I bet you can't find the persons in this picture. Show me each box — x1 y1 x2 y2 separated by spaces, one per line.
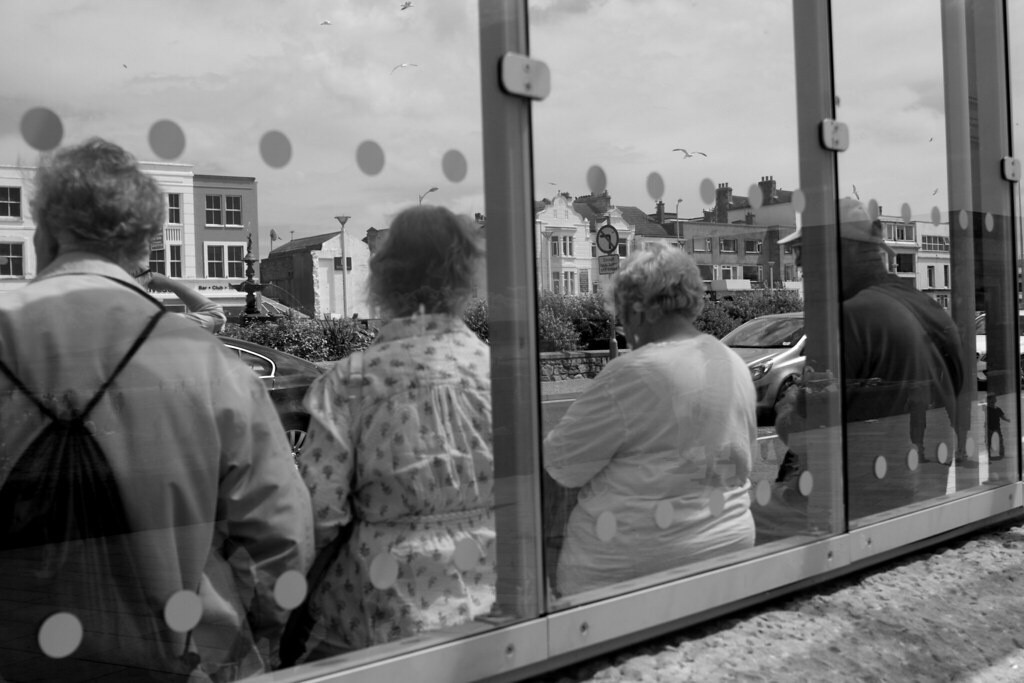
894 332 1011 462
747 200 965 545
542 240 761 597
272 203 496 674
0 135 315 683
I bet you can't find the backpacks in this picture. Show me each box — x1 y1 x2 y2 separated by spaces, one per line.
0 308 183 683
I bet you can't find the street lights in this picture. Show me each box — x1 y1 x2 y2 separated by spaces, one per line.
678 240 686 249
675 199 682 248
766 262 775 299
334 216 352 318
418 186 438 205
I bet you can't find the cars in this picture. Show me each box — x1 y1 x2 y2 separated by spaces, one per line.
217 334 328 471
976 311 1024 390
719 311 807 426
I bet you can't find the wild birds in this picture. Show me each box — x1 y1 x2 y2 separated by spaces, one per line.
123 1 708 185
852 137 939 201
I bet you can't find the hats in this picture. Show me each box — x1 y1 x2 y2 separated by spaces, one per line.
777 197 885 247
